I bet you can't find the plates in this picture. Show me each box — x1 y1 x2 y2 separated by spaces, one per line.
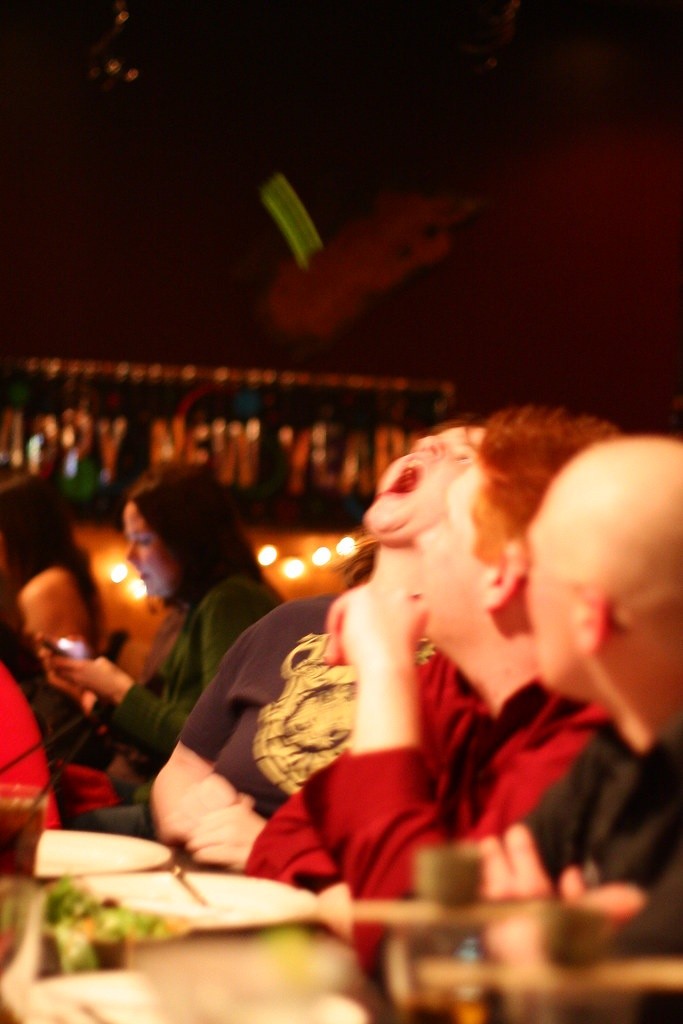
82 871 317 933
32 829 172 881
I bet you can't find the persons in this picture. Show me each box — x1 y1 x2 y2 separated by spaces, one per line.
1 467 280 864
472 433 682 1024
149 409 494 869
244 412 622 971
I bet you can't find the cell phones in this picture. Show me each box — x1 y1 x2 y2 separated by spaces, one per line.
42 638 65 658
